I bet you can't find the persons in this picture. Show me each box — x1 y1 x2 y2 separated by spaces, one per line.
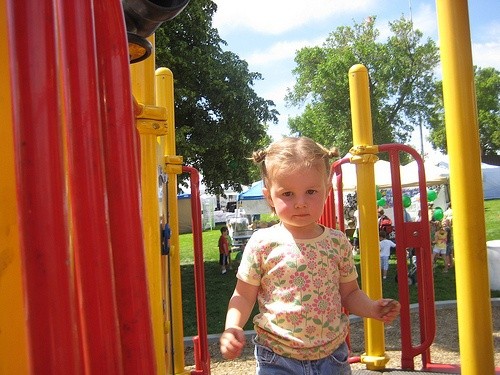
431 202 454 273
218 227 233 275
219 137 400 375
378 229 397 280
377 207 393 239
351 204 360 256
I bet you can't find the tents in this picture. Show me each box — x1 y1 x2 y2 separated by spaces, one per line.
374 152 500 211
328 153 402 221
237 180 269 213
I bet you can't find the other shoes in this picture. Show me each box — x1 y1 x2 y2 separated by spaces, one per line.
222 269 227 274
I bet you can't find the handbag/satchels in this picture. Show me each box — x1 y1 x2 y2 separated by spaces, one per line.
380 217 393 233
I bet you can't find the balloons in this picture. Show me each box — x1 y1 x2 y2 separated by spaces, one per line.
375 185 387 207
433 208 443 220
402 195 411 208
427 189 437 201
